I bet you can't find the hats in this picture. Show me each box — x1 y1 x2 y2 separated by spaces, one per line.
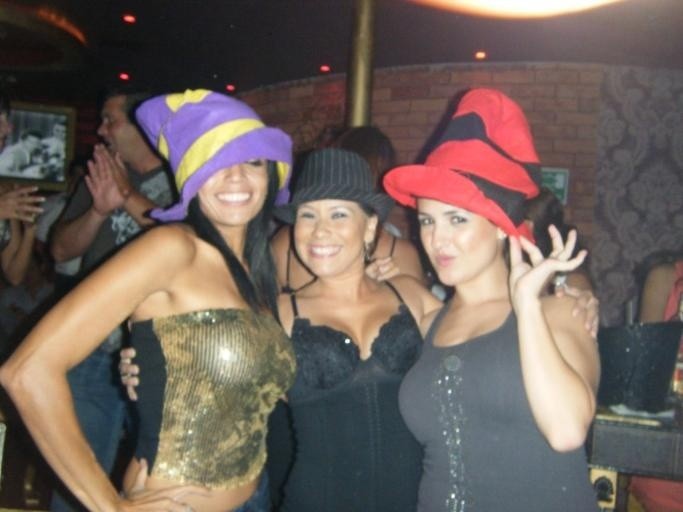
129 87 291 226
267 146 395 224
379 83 543 253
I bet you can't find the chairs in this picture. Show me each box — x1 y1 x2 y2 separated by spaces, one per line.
587 251 683 512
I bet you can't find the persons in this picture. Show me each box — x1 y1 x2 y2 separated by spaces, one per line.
628 257 683 512
0 87 601 510
